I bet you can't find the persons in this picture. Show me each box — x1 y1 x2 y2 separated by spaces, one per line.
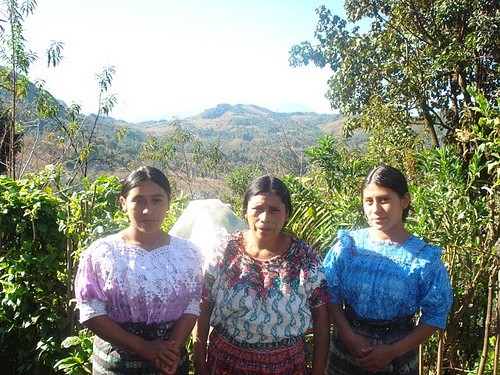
322 165 454 375
194 175 331 375
74 166 204 375
